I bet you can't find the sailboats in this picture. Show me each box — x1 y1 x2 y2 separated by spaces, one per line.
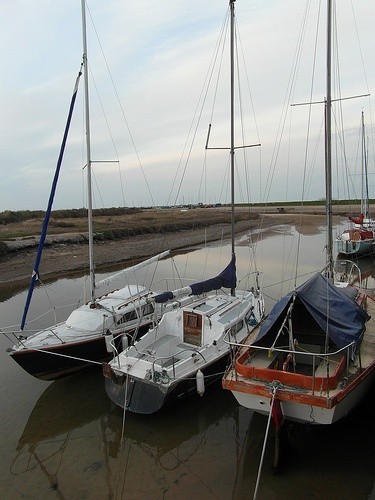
0 0 189 380
217 0 375 424
86 0 265 414
334 110 374 256
